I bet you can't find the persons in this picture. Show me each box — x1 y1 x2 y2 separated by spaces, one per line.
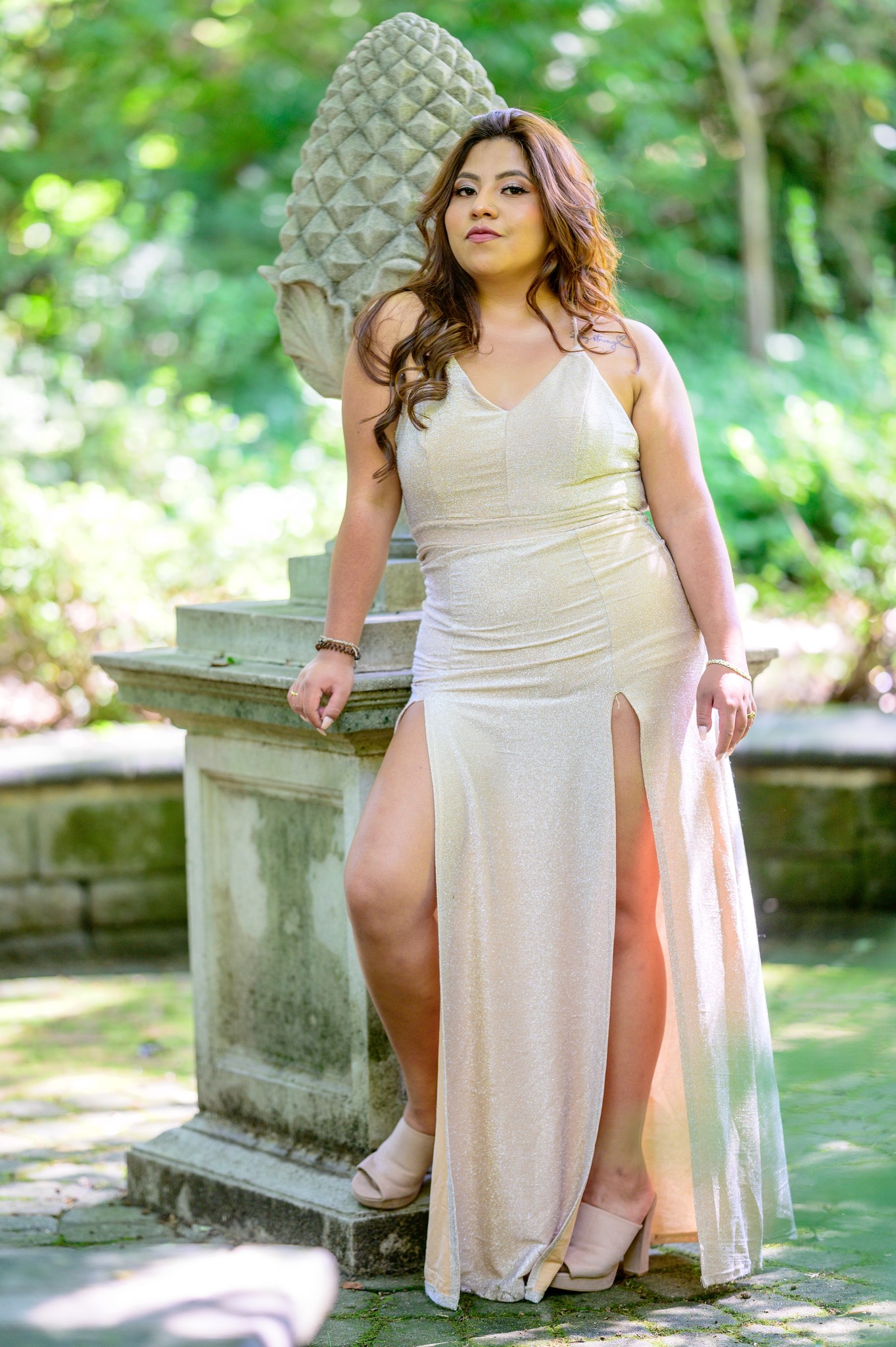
289 103 794 1306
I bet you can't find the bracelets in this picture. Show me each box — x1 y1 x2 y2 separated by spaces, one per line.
705 657 755 682
313 636 364 665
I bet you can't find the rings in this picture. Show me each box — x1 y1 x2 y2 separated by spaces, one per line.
744 710 759 721
292 691 301 698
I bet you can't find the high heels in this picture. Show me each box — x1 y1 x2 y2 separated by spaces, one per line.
351 1115 436 1209
552 1192 657 1292
